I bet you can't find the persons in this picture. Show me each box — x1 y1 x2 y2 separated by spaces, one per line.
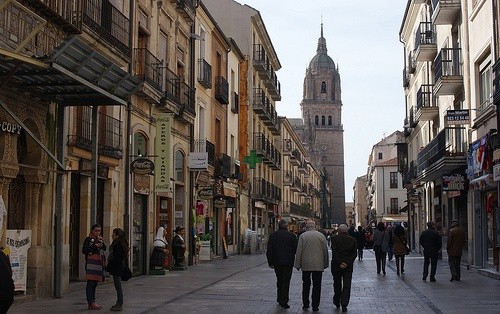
297 223 365 262
403 222 410 247
0 194 15 314
386 222 393 261
331 224 358 312
266 220 298 308
419 221 442 282
294 221 329 312
172 225 186 267
105 228 130 311
153 226 169 270
445 218 465 282
391 225 407 275
82 224 107 310
373 221 390 275
225 212 233 245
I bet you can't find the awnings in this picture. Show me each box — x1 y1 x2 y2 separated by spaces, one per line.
399 204 414 212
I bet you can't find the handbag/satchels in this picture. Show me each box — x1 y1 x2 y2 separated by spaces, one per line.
374 244 382 252
121 268 132 281
405 247 409 255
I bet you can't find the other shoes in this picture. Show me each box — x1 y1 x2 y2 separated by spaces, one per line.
422 277 426 280
430 278 437 282
110 305 123 311
456 278 460 281
342 307 347 312
333 300 340 308
397 272 399 275
278 301 290 308
377 272 380 274
383 271 386 274
88 303 102 310
313 307 320 311
450 274 457 282
401 270 404 273
303 305 309 309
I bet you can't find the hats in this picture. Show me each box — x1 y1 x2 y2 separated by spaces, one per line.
451 219 458 222
